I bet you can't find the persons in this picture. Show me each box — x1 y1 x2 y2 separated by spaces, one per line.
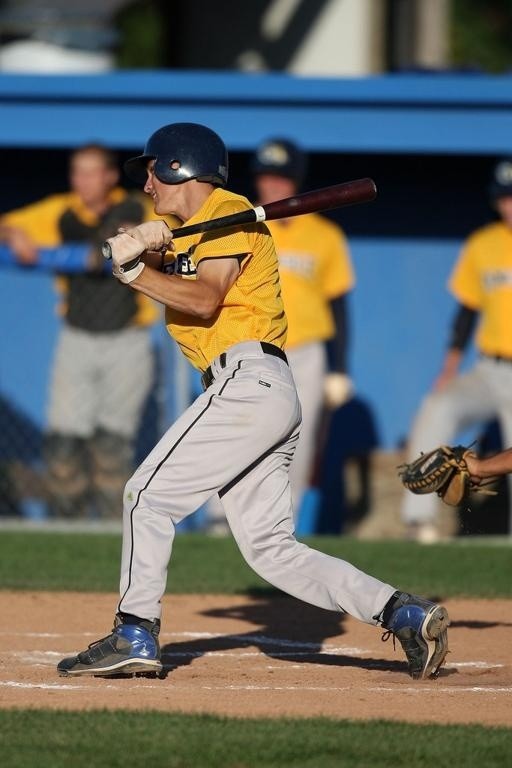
253 137 356 528
0 143 181 523
56 123 451 681
462 446 512 485
403 163 512 545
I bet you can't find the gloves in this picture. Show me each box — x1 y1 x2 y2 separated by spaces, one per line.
106 220 176 285
325 373 354 412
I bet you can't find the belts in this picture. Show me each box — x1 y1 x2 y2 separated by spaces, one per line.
200 341 288 391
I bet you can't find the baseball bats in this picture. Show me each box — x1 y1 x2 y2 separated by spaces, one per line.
101 176 377 259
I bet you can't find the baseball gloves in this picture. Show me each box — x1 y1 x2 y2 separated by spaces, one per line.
402 445 482 506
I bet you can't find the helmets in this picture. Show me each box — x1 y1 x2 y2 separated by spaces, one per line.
489 159 512 195
127 122 231 185
250 137 307 182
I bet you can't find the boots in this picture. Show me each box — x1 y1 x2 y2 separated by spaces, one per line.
57 614 165 678
376 590 449 681
